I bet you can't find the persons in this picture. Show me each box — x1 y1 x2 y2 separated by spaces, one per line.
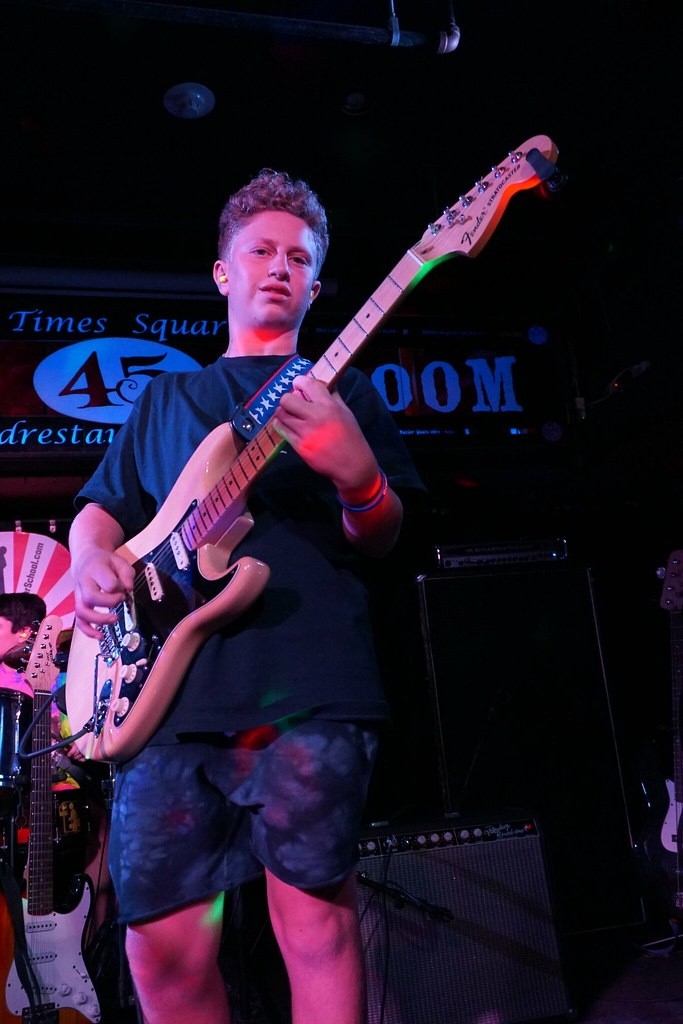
0 593 109 898
69 168 403 1024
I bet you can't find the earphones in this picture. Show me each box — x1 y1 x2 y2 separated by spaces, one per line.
218 276 227 283
310 291 314 297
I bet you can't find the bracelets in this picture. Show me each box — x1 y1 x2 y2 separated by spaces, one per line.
337 469 387 512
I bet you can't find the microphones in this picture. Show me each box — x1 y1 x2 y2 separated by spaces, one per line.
427 905 455 923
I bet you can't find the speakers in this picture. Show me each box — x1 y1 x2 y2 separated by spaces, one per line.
420 563 646 941
356 816 571 1024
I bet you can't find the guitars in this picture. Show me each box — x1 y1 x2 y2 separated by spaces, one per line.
4 609 117 1022
62 126 571 767
